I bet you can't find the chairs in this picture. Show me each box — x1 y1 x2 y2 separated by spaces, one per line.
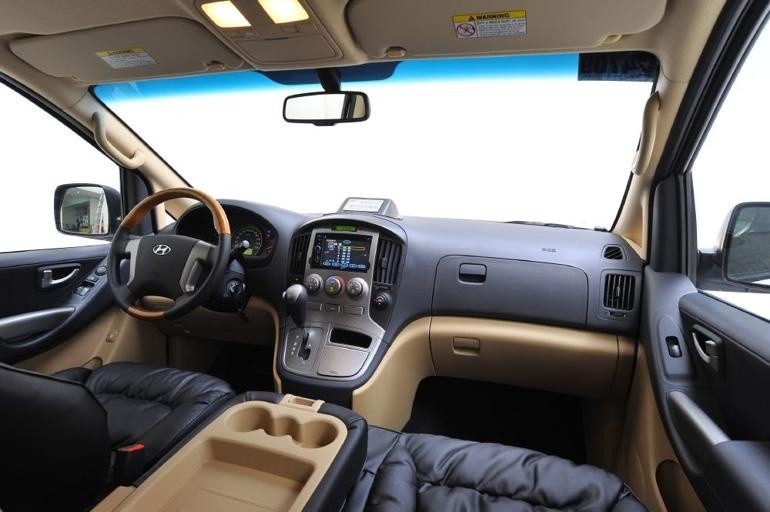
345 427 653 511
0 354 234 511
